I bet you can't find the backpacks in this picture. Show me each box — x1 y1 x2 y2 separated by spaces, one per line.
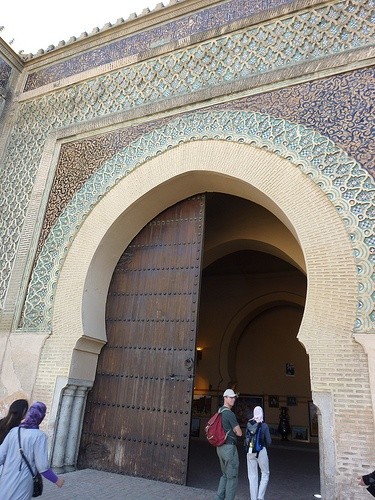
205 408 232 446
244 422 265 452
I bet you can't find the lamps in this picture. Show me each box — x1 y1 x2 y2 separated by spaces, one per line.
197 351 202 365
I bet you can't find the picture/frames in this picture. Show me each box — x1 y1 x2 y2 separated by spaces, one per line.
234 395 265 428
268 423 282 440
190 418 200 437
308 400 318 437
268 395 279 408
290 425 308 440
287 396 298 406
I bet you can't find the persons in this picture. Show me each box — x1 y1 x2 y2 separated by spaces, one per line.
246 406 272 500
0 399 65 500
357 470 375 498
216 389 242 500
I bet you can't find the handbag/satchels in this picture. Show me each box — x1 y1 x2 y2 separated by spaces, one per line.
33 473 43 497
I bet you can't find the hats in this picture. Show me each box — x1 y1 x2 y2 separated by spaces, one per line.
224 389 238 398
